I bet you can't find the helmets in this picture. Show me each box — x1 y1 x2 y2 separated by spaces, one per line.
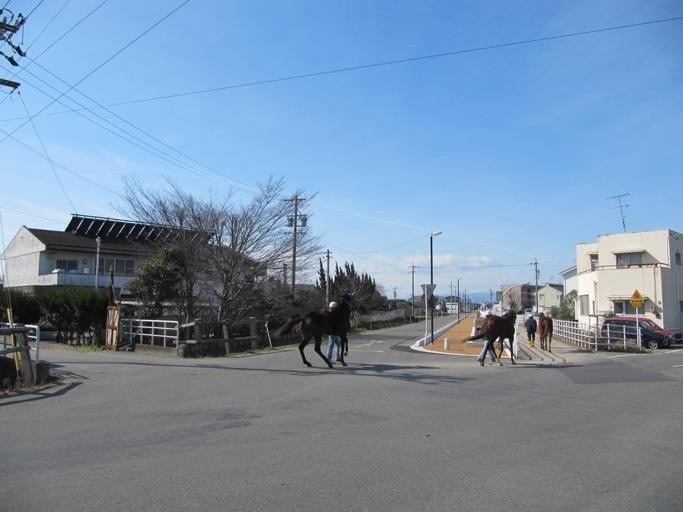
486 311 492 315
528 314 533 317
329 302 338 308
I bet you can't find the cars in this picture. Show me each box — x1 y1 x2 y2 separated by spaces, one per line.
601 316 676 350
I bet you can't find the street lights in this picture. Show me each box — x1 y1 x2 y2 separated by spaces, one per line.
429 230 442 344
456 276 464 324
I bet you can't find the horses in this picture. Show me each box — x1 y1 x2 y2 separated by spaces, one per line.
274 291 355 368
460 309 517 366
537 312 554 352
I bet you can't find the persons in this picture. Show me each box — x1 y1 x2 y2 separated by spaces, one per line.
476 310 501 367
523 315 537 348
325 301 344 365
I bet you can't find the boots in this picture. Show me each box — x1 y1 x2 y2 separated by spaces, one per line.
528 340 534 348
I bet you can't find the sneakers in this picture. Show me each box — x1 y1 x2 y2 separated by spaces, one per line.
329 360 335 364
335 357 341 361
